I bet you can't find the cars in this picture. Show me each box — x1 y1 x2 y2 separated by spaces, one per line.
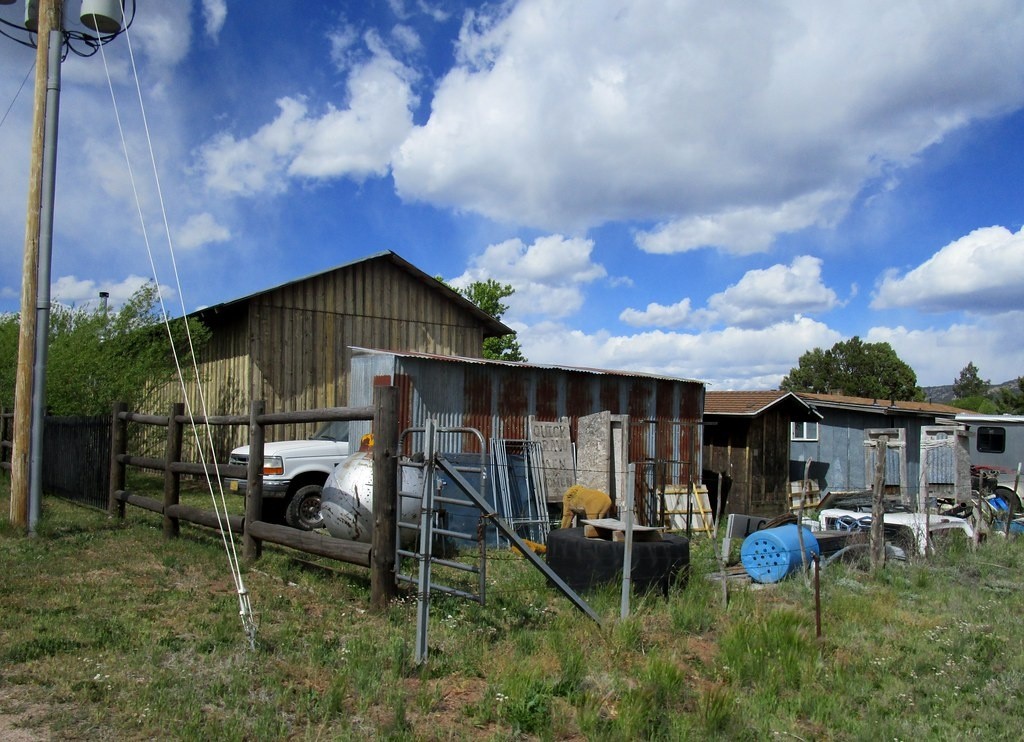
224 421 351 531
